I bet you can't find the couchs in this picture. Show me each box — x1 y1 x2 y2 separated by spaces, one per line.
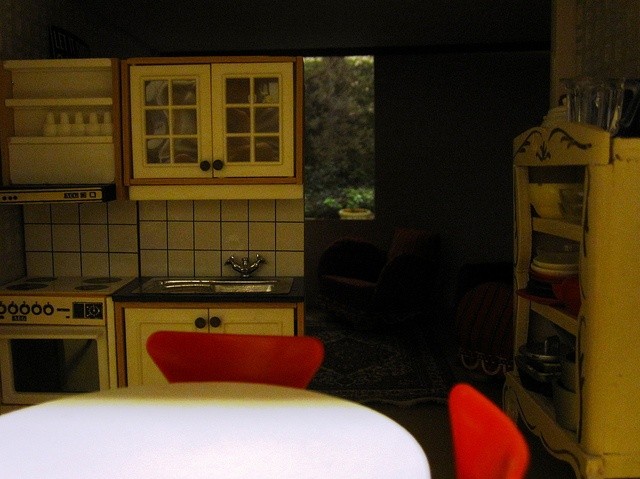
317 226 441 329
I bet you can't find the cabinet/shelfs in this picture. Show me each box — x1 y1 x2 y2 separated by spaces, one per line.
502 122 640 479
114 302 305 389
3 58 116 184
120 55 304 187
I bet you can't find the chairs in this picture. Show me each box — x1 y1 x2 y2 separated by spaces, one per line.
447 383 531 479
146 331 325 389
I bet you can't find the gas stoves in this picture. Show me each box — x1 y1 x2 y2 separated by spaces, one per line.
0 274 130 327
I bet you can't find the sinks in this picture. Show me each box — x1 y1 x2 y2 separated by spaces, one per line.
129 278 212 294
214 277 294 294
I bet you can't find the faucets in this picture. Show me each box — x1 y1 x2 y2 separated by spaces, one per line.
240 258 252 276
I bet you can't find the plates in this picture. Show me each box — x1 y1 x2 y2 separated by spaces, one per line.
530 253 583 280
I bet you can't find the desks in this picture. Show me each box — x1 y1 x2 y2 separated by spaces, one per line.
0 382 432 479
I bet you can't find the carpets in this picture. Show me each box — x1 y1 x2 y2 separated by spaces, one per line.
304 319 493 407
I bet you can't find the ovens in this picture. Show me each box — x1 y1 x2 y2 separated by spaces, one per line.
1 324 110 407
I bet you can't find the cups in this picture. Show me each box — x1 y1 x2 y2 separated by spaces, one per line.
559 75 639 137
